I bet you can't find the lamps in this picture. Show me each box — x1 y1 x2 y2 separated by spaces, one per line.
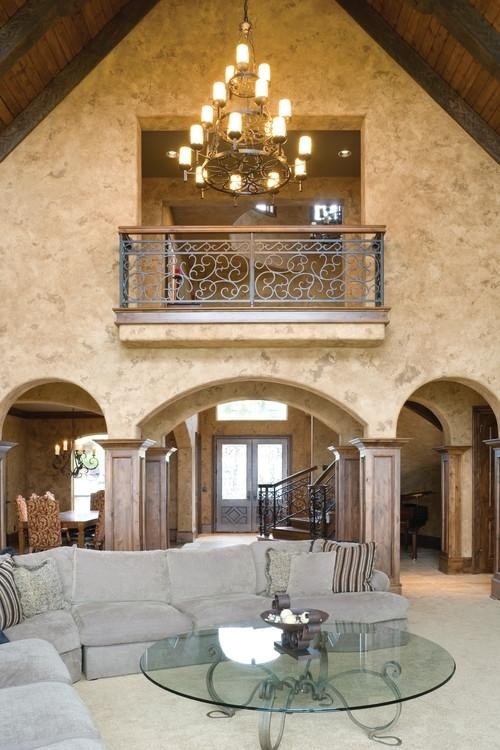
177 0 319 205
51 408 98 480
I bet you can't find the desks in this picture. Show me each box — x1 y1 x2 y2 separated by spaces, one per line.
139 624 456 747
14 484 116 554
